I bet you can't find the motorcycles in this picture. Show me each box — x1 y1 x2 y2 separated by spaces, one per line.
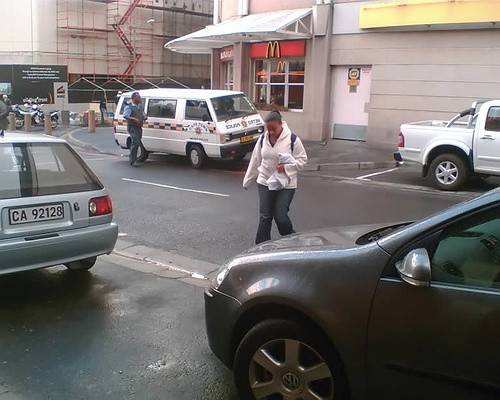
7 104 43 129
28 106 59 129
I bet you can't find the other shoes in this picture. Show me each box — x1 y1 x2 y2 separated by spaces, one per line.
131 162 140 167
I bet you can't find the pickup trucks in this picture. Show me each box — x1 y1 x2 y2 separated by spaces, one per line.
394 99 500 191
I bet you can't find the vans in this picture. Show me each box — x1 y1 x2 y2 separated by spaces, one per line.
112 88 267 169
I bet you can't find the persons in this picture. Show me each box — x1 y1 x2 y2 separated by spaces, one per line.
123 92 147 167
0 94 11 135
100 91 122 126
160 103 175 118
242 111 308 245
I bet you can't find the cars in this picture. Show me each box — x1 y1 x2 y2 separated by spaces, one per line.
0 130 119 277
203 186 500 399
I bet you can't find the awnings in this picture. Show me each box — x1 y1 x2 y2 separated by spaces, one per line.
163 9 313 54
